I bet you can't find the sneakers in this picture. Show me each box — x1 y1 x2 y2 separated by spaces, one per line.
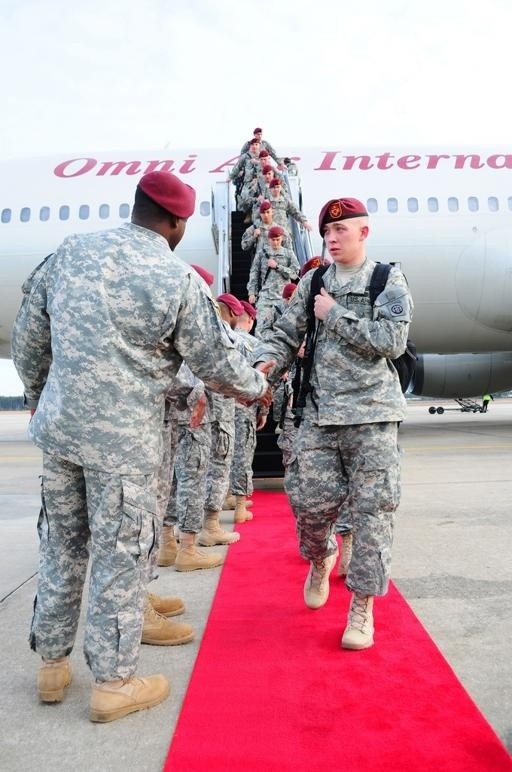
36 655 73 705
147 589 187 618
88 675 169 724
139 601 196 646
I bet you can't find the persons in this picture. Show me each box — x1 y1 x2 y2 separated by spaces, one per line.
11 169 272 721
139 264 268 646
229 128 313 344
482 394 493 411
253 197 414 649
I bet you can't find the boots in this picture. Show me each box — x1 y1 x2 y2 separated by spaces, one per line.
302 552 337 610
198 508 240 546
174 530 226 571
234 494 253 524
340 590 375 649
275 421 283 436
338 534 353 576
157 525 179 567
221 490 253 511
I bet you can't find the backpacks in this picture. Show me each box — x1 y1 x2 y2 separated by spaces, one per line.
309 262 417 394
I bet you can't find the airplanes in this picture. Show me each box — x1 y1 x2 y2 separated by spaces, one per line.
0 140 512 482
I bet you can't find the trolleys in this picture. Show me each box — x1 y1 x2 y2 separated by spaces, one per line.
427 397 488 415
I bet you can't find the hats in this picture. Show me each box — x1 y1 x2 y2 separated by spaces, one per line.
262 165 274 176
318 197 368 238
190 264 214 286
139 170 195 219
259 202 271 214
250 138 260 145
282 283 296 299
216 294 243 316
258 150 269 158
268 226 284 239
269 178 281 189
239 300 256 320
253 128 263 134
300 256 331 279
